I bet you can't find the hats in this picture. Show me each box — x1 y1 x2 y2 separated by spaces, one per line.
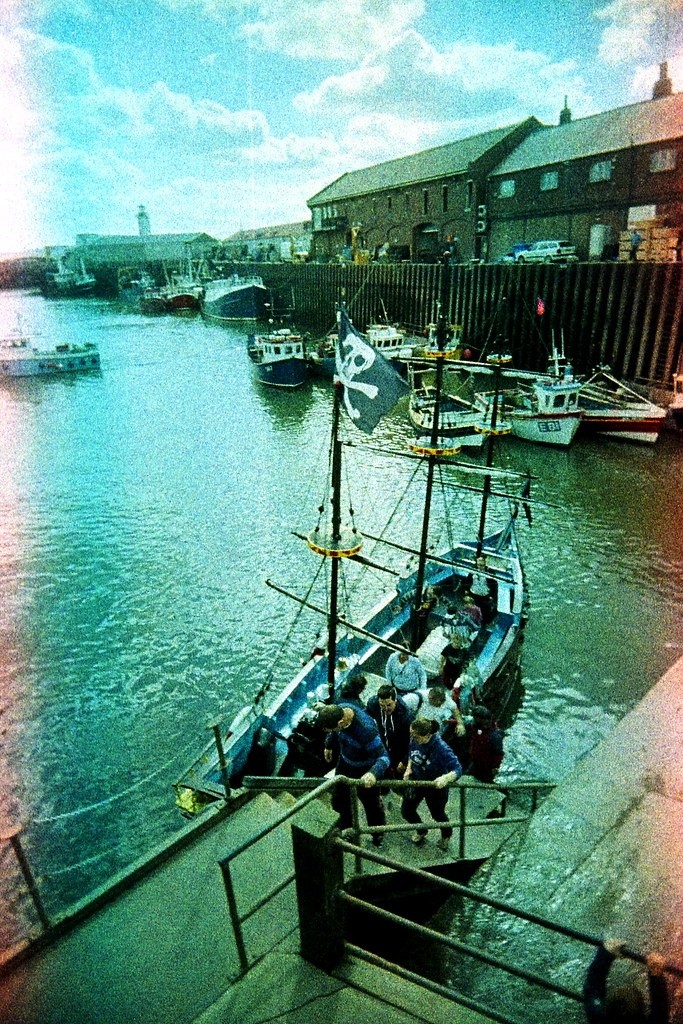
460 674 475 689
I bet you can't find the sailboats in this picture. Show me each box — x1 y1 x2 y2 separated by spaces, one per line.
170 251 531 802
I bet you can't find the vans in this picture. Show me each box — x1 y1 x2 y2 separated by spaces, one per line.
280 242 309 263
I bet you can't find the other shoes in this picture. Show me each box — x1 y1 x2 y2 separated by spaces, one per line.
411 831 425 843
333 819 351 831
372 834 383 847
439 836 450 849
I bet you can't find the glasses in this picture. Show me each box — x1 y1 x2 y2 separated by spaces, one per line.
463 600 470 604
477 564 485 566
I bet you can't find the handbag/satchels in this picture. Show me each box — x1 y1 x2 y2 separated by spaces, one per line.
406 774 418 798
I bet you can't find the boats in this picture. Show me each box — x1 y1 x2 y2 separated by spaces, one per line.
576 366 665 447
0 334 102 379
363 323 462 362
115 257 268 321
39 253 97 297
473 347 585 449
658 374 683 432
408 366 514 449
244 300 339 390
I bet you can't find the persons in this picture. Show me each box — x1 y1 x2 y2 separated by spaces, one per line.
317 702 390 848
400 718 462 850
629 227 643 263
366 583 503 789
466 556 496 630
441 234 458 264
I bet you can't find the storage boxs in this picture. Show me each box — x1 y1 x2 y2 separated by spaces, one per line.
619 216 683 261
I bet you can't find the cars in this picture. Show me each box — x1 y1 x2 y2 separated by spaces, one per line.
514 239 578 264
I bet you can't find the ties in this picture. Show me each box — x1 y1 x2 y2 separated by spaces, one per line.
386 714 393 748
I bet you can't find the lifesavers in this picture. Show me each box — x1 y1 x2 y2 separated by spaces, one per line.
453 675 477 714
